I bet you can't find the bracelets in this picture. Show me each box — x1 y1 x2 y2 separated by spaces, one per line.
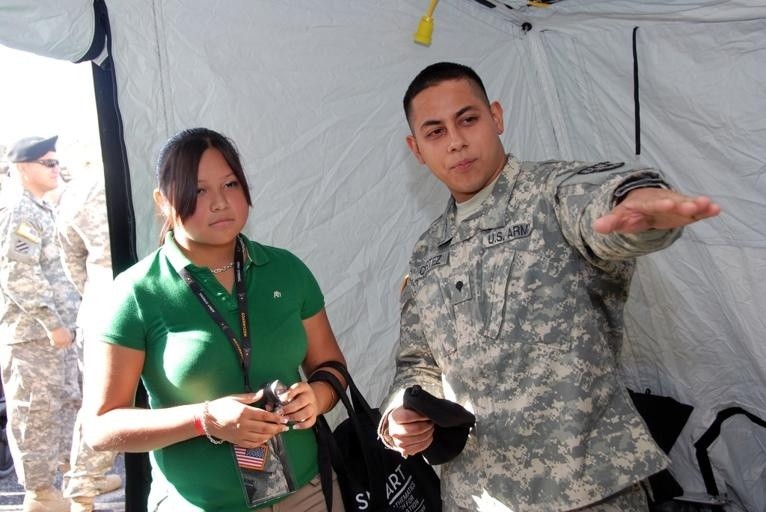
201 399 224 445
192 404 206 435
317 381 335 413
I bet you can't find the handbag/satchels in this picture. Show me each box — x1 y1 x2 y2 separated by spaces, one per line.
306 359 445 512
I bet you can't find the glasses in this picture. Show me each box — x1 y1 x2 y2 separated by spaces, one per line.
35 159 59 168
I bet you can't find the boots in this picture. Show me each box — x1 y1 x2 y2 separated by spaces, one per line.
22 484 94 512
61 472 122 499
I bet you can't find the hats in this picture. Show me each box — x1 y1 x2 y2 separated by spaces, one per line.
7 135 58 163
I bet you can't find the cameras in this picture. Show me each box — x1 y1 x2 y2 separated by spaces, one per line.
260 378 306 424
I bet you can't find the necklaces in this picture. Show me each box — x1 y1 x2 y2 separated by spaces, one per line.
209 235 246 275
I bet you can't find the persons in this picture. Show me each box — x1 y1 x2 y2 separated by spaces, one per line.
2 131 85 510
371 62 723 510
57 141 129 511
79 128 351 512
